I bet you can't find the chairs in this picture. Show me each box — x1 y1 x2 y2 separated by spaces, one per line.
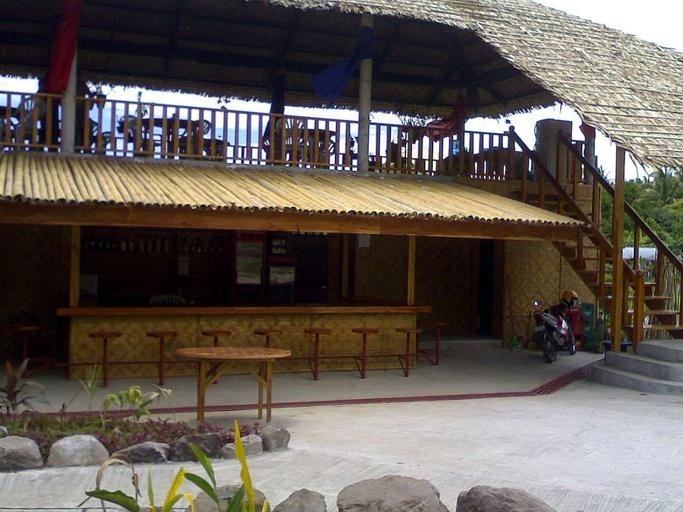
1 114 338 173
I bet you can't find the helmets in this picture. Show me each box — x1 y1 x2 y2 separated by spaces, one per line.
558 288 578 306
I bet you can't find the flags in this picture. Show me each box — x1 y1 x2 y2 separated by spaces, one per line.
39 1 80 109
311 25 374 103
421 94 466 141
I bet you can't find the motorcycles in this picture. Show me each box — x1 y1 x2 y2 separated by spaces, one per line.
531 300 576 364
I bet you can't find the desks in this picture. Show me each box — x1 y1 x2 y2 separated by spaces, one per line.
176 346 292 424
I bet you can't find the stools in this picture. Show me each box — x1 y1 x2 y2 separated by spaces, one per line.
253 329 282 382
146 330 178 386
352 328 379 379
421 318 447 365
202 329 231 384
19 325 42 376
89 331 122 387
304 328 332 380
397 327 423 377
